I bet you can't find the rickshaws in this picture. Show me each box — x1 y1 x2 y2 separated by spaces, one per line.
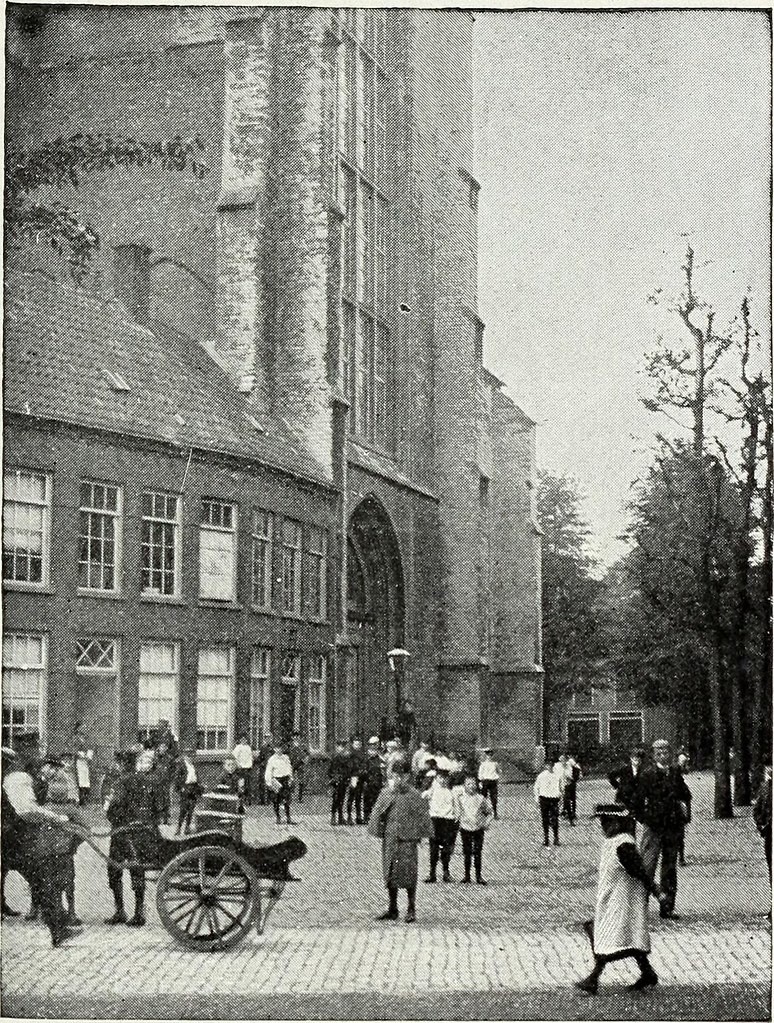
51 817 311 952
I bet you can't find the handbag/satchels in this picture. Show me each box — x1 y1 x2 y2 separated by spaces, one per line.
375 805 392 838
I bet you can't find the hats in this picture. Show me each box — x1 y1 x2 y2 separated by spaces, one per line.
391 759 412 774
651 738 669 749
544 755 553 763
11 730 40 749
367 735 380 744
589 802 630 818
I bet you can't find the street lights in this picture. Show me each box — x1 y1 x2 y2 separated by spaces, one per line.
385 643 412 741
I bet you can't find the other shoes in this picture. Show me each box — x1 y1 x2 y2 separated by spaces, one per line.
627 970 659 990
574 979 599 996
52 926 85 948
442 874 456 882
405 910 415 923
124 913 146 925
477 877 487 885
424 876 436 882
103 910 128 924
459 877 472 884
376 910 399 920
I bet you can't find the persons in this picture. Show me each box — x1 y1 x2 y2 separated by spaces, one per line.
532 752 584 845
330 737 500 922
103 752 154 924
752 769 773 921
121 720 309 837
1 720 93 946
575 737 692 994
